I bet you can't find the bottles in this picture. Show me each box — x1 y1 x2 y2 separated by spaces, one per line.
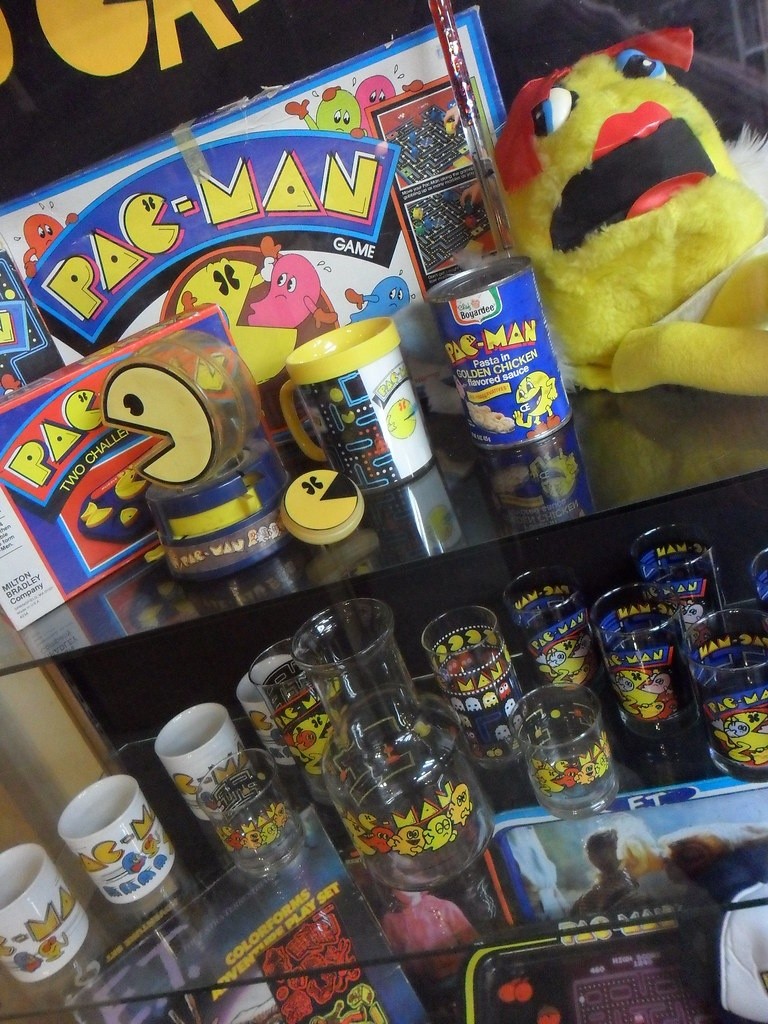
323 687 490 891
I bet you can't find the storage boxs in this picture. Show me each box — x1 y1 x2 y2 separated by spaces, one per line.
1 302 277 632
0 6 515 451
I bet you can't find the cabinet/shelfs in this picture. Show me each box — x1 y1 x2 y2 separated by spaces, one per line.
0 389 767 1023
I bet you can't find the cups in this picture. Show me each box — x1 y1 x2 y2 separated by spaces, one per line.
237 655 319 765
508 681 618 822
588 581 699 740
196 748 301 881
277 316 432 497
504 566 601 699
684 548 768 784
154 702 256 823
629 523 726 667
56 775 173 904
0 843 89 984
421 606 528 769
249 605 413 806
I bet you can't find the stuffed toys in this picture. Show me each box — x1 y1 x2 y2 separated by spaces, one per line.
494 29 768 402
